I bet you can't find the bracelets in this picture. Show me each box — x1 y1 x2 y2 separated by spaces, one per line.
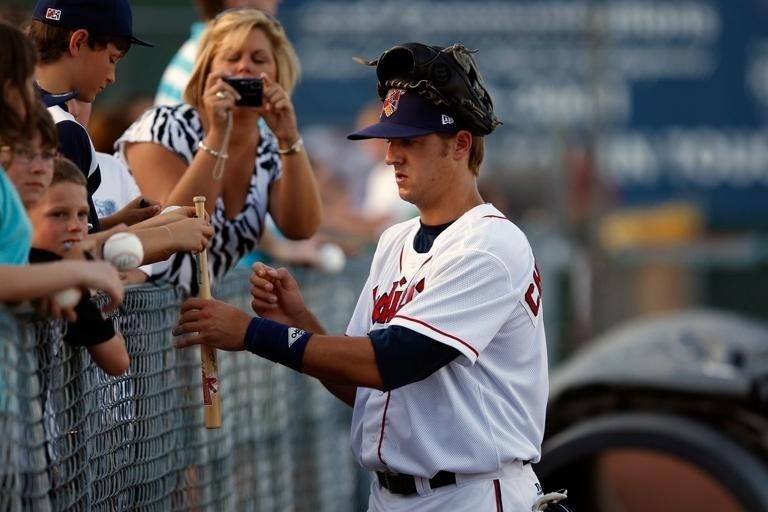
241 317 313 374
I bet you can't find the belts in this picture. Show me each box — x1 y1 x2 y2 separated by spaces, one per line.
375 470 455 496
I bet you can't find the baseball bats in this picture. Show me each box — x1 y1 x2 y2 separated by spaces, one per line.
192 195 225 427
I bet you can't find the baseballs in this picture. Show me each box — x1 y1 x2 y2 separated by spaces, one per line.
103 231 145 272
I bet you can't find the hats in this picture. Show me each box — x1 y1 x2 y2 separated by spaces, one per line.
346 87 483 140
31 0 154 47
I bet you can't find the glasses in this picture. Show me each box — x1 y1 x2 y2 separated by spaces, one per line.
0 144 60 169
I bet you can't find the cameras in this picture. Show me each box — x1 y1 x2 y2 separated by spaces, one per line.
222 79 262 107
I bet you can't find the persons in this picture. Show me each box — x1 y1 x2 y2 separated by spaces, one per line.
2 1 417 381
167 90 553 512
